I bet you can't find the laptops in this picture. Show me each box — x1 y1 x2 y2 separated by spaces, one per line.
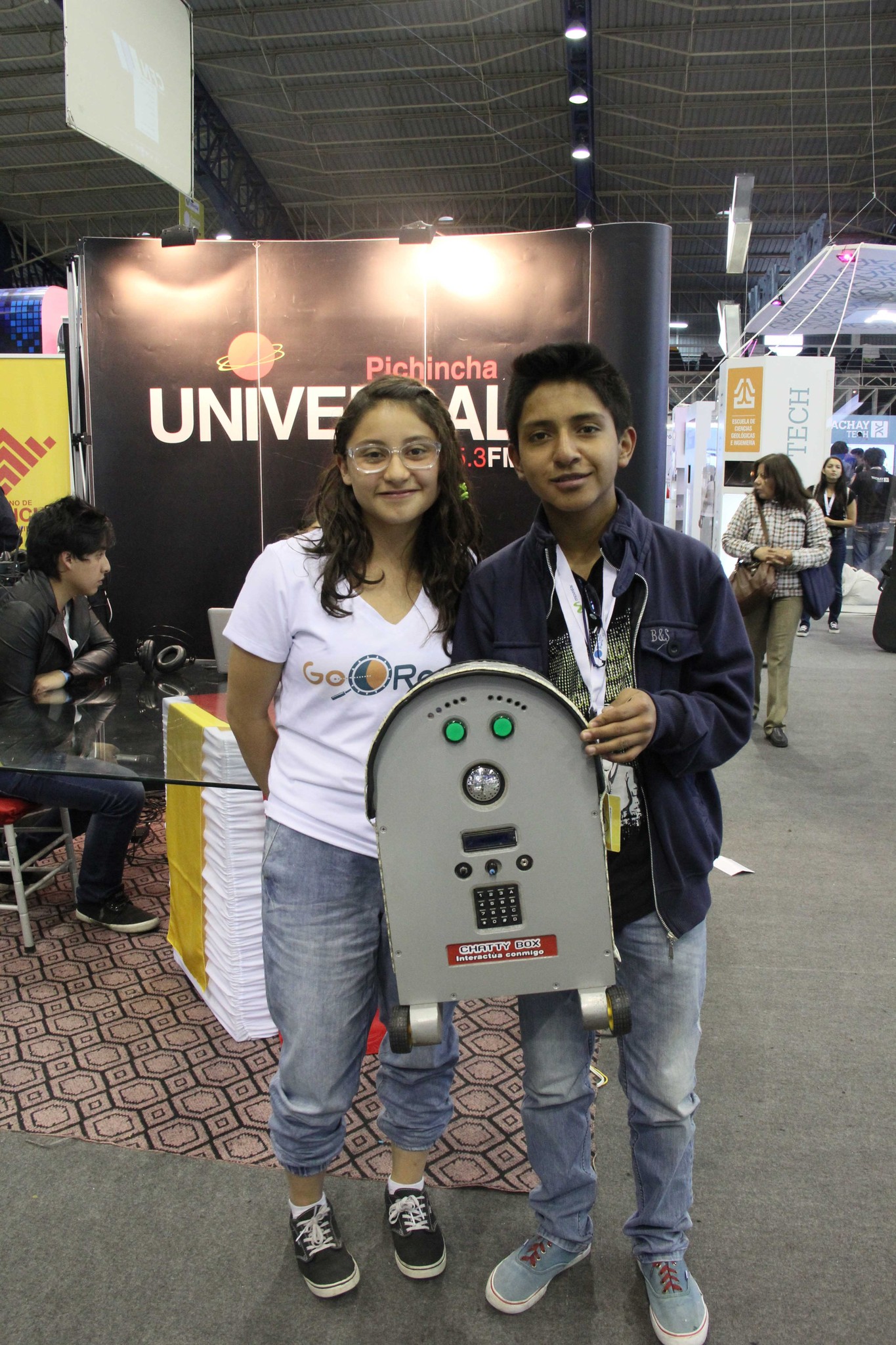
208 608 233 674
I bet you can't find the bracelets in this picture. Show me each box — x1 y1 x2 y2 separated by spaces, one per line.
58 667 72 683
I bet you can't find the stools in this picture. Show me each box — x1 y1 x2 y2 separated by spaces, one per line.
0 792 82 954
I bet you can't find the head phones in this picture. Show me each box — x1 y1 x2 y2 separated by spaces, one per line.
134 625 197 676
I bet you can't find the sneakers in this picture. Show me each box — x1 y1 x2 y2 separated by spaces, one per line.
485 1234 592 1314
828 621 839 633
290 1197 359 1297
76 885 160 932
0 873 54 891
796 625 809 637
384 1182 447 1278
636 1259 709 1345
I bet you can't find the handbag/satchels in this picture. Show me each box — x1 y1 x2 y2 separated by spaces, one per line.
729 559 777 617
797 563 837 620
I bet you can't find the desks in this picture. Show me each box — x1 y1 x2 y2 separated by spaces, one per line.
0 660 279 790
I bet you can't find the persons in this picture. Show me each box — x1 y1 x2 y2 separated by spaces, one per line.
720 453 833 747
224 374 512 1296
792 456 858 636
0 495 160 933
438 341 765 1344
829 438 895 583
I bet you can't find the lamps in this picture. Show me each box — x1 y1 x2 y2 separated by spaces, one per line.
576 208 592 228
136 224 150 236
837 249 858 264
771 295 786 306
161 225 198 248
567 75 588 105
564 11 586 40
214 221 232 241
571 129 590 159
399 220 436 244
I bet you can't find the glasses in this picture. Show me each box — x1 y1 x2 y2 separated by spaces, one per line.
343 439 444 474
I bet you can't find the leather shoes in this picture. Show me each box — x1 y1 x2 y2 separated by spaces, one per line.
767 727 788 747
